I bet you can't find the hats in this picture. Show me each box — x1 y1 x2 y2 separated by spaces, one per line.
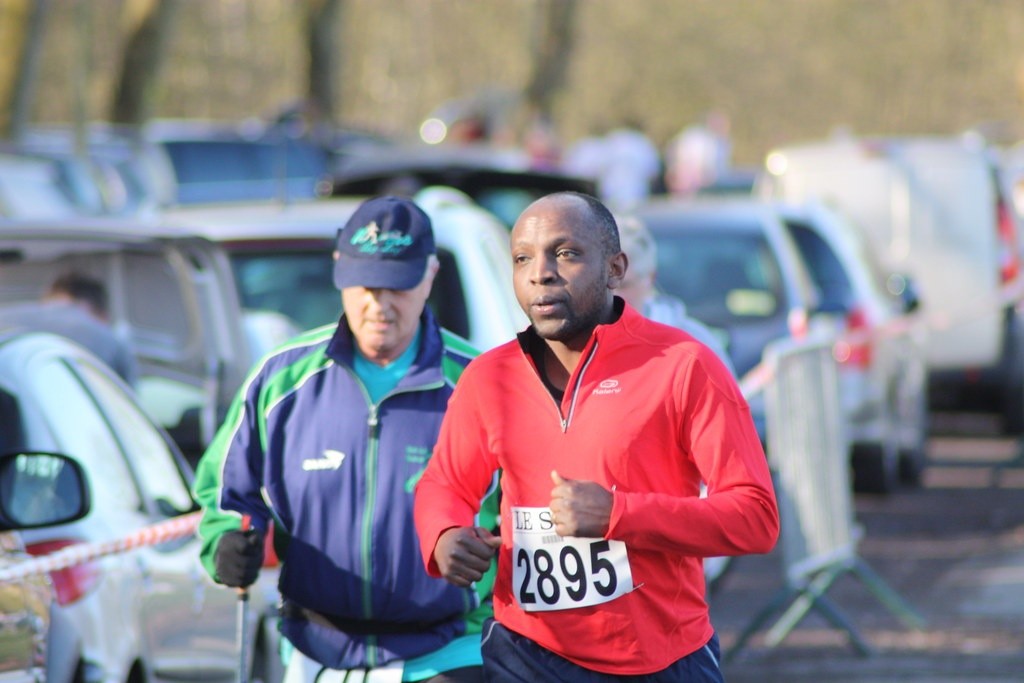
332 194 436 290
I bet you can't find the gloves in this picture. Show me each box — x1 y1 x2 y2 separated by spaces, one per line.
215 530 264 589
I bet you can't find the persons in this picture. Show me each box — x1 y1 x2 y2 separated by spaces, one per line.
413 191 781 683
0 258 130 386
191 193 501 683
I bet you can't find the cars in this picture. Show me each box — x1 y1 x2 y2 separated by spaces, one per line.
0 106 1024 493
0 330 291 683
